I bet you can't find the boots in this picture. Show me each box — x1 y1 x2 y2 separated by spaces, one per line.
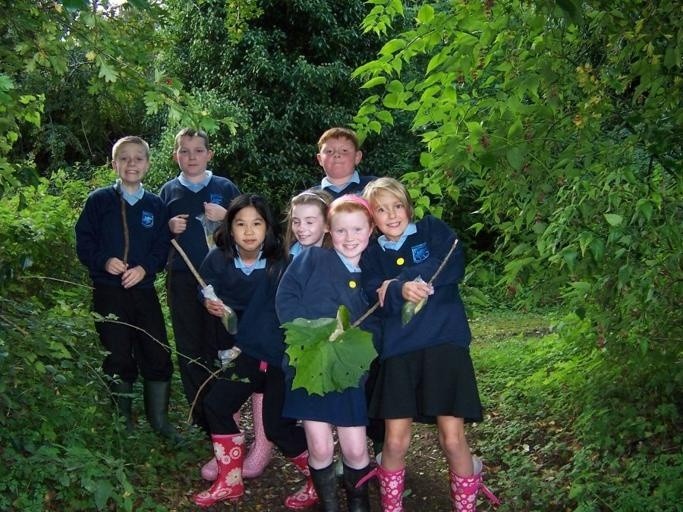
356 452 408 512
307 456 341 512
342 454 371 512
194 428 246 507
240 392 274 479
284 451 323 510
112 379 136 434
200 409 241 481
448 457 497 512
142 379 181 443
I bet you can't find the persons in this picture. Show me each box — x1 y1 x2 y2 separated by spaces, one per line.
304 127 385 482
159 126 240 438
194 192 286 479
75 134 180 445
361 176 482 512
194 188 337 507
275 192 378 512
